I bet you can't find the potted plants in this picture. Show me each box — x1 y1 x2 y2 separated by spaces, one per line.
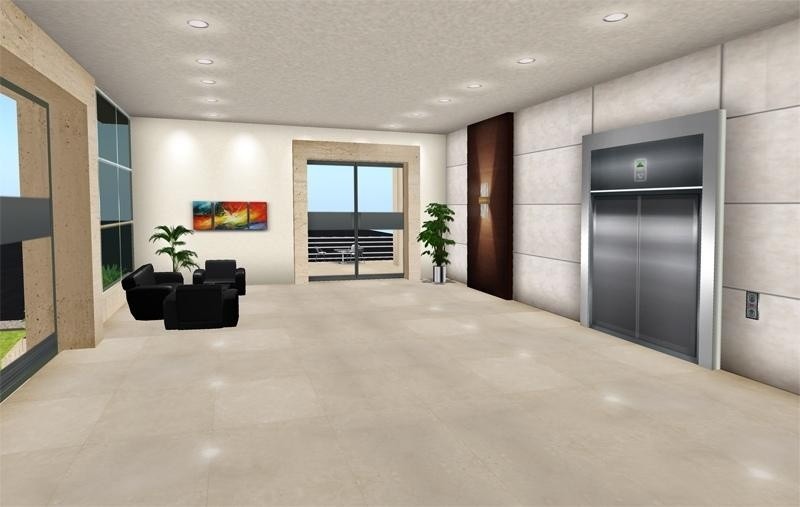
416 202 457 284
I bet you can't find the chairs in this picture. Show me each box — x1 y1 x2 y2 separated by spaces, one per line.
121 259 247 329
